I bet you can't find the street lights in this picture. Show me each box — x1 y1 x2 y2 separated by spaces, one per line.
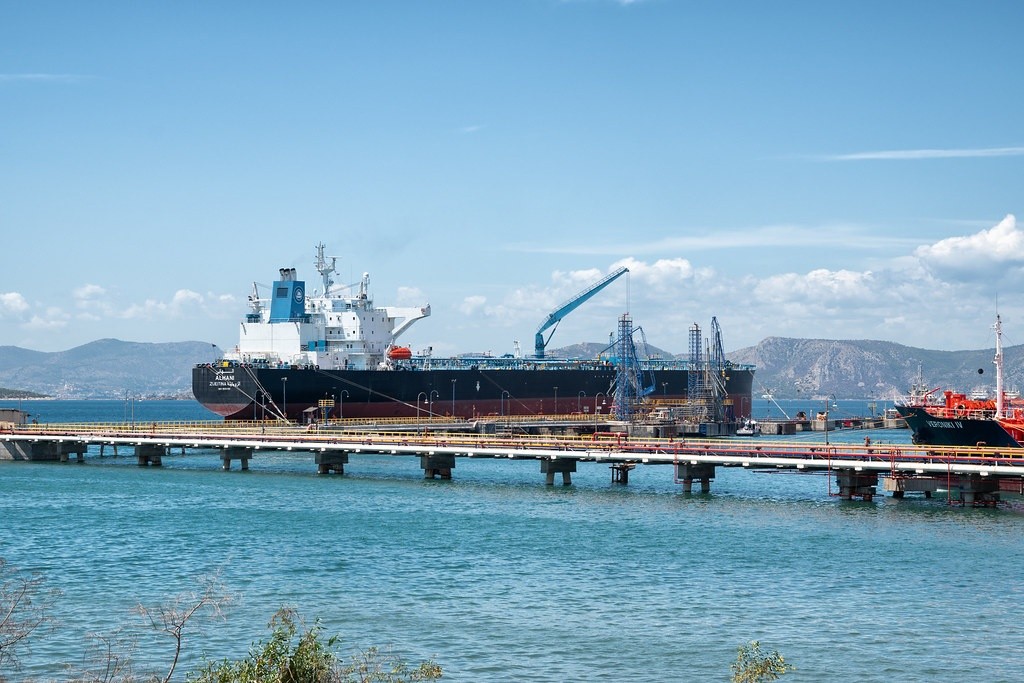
451 379 457 416
870 390 874 418
594 392 607 441
131 392 143 426
261 393 273 442
123 389 135 422
553 387 559 416
824 393 839 449
417 392 429 437
281 376 287 418
429 390 439 418
253 389 267 423
340 389 349 419
500 390 510 416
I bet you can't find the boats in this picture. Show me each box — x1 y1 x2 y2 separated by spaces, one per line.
895 291 1024 450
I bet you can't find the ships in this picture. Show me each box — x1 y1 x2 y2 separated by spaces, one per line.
190 241 759 423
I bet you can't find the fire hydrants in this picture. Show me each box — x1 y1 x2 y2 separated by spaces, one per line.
577 391 586 412
